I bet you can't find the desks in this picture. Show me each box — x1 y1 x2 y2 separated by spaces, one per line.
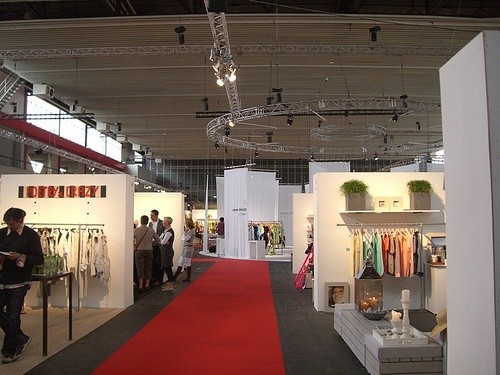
334 303 444 375
31 271 74 356
423 261 448 315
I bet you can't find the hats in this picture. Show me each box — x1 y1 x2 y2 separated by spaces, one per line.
431 309 447 337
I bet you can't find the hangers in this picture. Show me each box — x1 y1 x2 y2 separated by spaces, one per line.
24 222 105 237
247 221 283 227
349 222 420 239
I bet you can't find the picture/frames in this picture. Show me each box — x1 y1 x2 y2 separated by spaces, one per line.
324 281 349 313
374 196 389 211
389 196 403 211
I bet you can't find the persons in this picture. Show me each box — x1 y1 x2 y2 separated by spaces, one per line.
158 217 174 291
173 219 194 282
218 217 224 236
0 208 44 363
195 226 203 244
149 209 165 238
134 215 158 292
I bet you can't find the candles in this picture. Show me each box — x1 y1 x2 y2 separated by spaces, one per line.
362 302 368 309
371 296 378 310
391 310 402 320
401 289 410 300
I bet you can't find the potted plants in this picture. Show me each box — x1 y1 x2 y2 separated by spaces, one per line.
406 179 433 210
337 179 369 211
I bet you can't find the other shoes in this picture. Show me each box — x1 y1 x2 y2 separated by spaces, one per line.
161 286 173 291
153 281 160 285
17 337 32 360
145 287 150 292
182 278 192 282
138 287 144 293
1 354 17 363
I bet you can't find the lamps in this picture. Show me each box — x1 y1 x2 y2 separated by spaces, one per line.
0 8 433 209
